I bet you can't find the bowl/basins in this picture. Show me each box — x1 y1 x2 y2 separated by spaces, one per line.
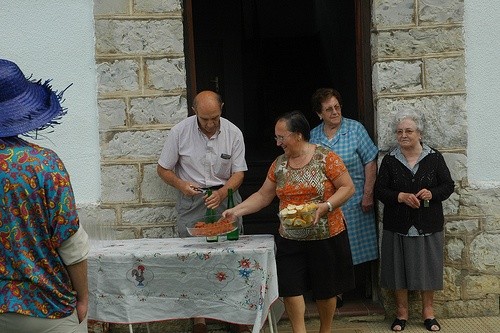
280 208 315 229
185 214 239 236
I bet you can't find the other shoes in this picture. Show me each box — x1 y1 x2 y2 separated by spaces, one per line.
193 323 208 333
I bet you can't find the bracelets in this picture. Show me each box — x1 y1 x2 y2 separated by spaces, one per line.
326 200 334 213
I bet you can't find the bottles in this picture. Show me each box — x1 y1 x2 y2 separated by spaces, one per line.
225 188 239 240
205 190 219 242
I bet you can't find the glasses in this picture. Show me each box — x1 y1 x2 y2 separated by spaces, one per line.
324 104 343 113
396 128 419 135
274 132 293 142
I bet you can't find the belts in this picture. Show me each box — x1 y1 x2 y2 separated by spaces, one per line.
201 185 224 191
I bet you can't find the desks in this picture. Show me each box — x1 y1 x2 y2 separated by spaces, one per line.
87 235 279 333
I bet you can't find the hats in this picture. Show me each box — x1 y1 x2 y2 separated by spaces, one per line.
0 59 74 139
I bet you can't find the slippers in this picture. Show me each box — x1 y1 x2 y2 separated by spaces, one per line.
391 318 408 331
424 318 442 331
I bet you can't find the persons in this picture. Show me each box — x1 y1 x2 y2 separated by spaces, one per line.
373 107 455 331
1 59 90 333
309 89 381 310
157 91 248 237
222 111 355 333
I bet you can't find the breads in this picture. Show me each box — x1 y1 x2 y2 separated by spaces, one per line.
279 203 319 228
